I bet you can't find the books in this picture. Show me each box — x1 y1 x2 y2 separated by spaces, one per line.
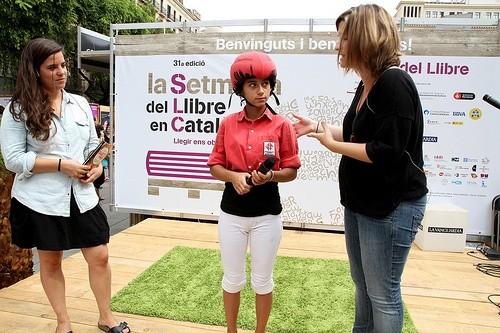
81 141 112 172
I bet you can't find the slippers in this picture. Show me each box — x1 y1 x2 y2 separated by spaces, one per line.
98 322 130 333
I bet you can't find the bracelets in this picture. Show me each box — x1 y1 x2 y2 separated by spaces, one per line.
316 121 320 133
58 159 61 172
268 170 274 182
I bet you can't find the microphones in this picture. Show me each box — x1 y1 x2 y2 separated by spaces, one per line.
483 94 500 109
246 158 276 185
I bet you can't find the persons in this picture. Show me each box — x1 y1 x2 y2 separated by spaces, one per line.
292 3 429 333
206 50 301 333
0 38 131 333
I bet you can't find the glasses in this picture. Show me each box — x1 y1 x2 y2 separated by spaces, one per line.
336 35 347 44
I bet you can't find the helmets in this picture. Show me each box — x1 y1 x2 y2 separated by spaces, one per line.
230 51 277 96
